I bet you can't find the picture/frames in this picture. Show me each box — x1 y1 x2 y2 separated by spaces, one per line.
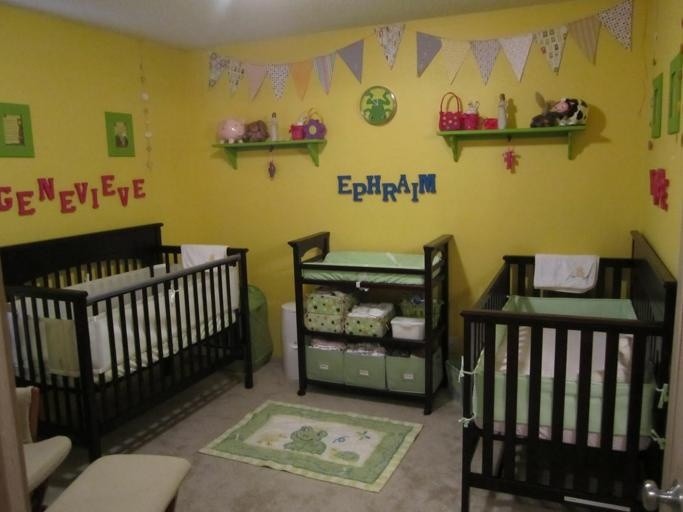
0 103 35 157
651 72 663 137
105 112 135 157
667 52 682 133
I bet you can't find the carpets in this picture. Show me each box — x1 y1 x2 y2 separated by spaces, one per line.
198 399 425 493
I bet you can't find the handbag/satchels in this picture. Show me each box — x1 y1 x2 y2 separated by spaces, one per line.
438 90 464 131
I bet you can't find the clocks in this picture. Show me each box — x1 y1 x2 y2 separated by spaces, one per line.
360 85 397 126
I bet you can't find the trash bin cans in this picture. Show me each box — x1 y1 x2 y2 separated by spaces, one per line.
281 302 299 379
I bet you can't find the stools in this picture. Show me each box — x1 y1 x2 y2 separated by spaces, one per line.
42 454 194 512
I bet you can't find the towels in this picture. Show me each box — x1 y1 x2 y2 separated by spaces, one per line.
181 243 230 271
534 254 600 295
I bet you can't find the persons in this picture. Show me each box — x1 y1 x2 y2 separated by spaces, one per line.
269 111 278 141
495 93 509 131
113 121 129 147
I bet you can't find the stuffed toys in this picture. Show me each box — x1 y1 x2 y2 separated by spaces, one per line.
550 96 587 126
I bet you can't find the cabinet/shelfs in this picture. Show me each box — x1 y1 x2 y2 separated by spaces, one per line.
287 232 453 416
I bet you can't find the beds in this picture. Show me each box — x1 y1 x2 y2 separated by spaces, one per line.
0 223 253 463
459 230 678 512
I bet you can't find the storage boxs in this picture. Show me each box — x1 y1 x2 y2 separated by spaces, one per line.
302 294 444 396
